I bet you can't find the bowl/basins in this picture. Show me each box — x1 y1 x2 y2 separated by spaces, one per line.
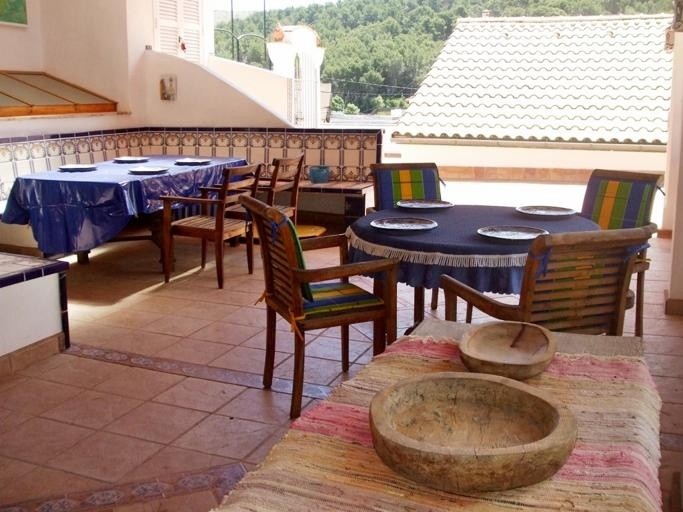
366 372 576 494
458 321 556 377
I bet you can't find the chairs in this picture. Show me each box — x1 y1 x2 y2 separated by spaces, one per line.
370 162 442 310
158 157 305 290
439 224 658 336
239 195 398 419
580 169 666 337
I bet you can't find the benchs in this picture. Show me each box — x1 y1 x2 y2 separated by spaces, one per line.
0 128 382 383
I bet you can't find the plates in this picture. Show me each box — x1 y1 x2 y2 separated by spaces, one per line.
368 217 438 234
515 205 577 219
174 156 211 167
59 164 97 172
475 225 548 242
126 167 170 173
395 199 455 211
112 156 152 162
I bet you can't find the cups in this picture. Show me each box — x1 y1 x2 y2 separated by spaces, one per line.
310 165 328 183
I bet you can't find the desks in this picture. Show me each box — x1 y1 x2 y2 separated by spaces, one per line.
209 313 663 511
0 154 250 282
1 250 70 350
